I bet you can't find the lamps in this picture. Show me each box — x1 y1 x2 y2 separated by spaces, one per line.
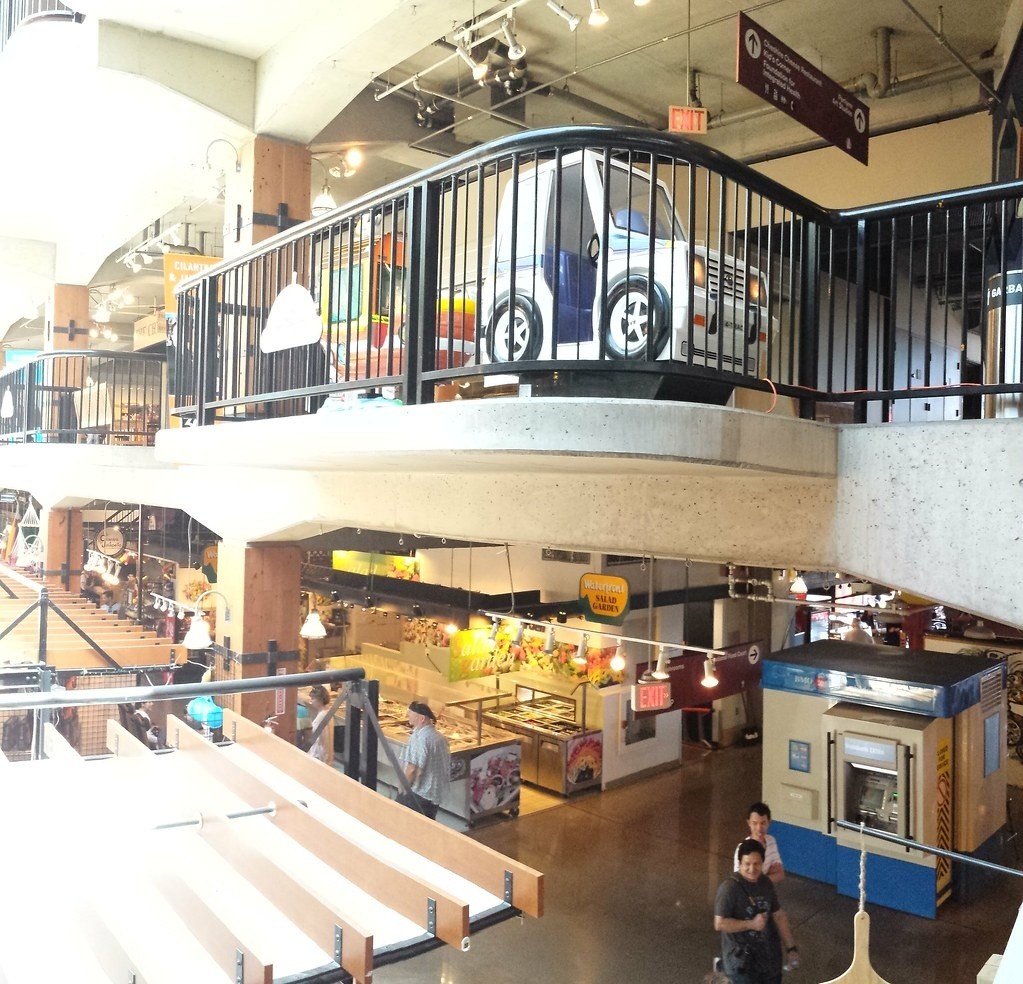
170 232 182 246
588 0 609 26
455 46 488 80
129 260 142 273
329 588 342 603
140 252 153 264
310 157 337 217
363 594 375 609
156 241 170 254
83 552 185 619
183 589 229 650
502 22 526 60
484 618 720 688
301 586 327 640
790 570 809 594
410 603 426 619
547 0 583 32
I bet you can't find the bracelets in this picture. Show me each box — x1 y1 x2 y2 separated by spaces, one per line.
787 945 798 952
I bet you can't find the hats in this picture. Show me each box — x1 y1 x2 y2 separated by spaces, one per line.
409 701 437 721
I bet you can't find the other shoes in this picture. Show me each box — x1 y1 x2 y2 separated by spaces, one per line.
712 956 723 976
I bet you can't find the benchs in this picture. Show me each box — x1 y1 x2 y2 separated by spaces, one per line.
544 245 596 344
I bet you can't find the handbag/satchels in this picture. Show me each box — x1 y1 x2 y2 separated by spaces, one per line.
329 712 346 753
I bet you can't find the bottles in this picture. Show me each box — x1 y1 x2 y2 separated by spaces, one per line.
783 957 802 972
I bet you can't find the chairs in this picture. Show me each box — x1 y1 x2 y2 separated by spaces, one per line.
73 381 148 446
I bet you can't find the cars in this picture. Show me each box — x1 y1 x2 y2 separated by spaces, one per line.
472 151 780 366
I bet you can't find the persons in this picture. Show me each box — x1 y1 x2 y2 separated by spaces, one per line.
305 682 333 767
846 604 906 648
732 802 786 884
714 839 805 984
134 700 159 745
392 698 449 821
99 590 122 613
